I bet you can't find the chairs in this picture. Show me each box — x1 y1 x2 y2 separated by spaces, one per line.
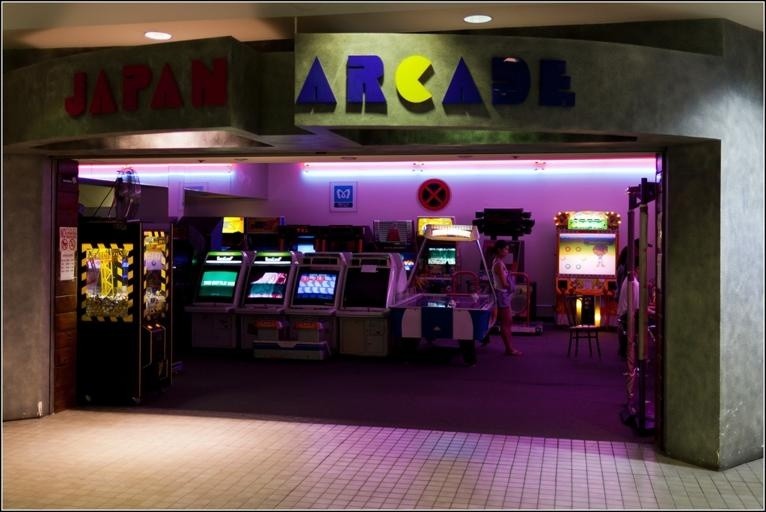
567 294 603 360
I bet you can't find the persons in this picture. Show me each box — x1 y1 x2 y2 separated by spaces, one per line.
480 240 529 356
614 238 657 358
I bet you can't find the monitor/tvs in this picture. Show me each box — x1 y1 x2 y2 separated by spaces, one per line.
244 266 290 305
290 264 344 310
339 265 394 311
196 265 241 303
296 242 316 253
426 247 456 266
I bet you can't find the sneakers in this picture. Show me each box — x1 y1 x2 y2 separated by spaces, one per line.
504 350 523 356
